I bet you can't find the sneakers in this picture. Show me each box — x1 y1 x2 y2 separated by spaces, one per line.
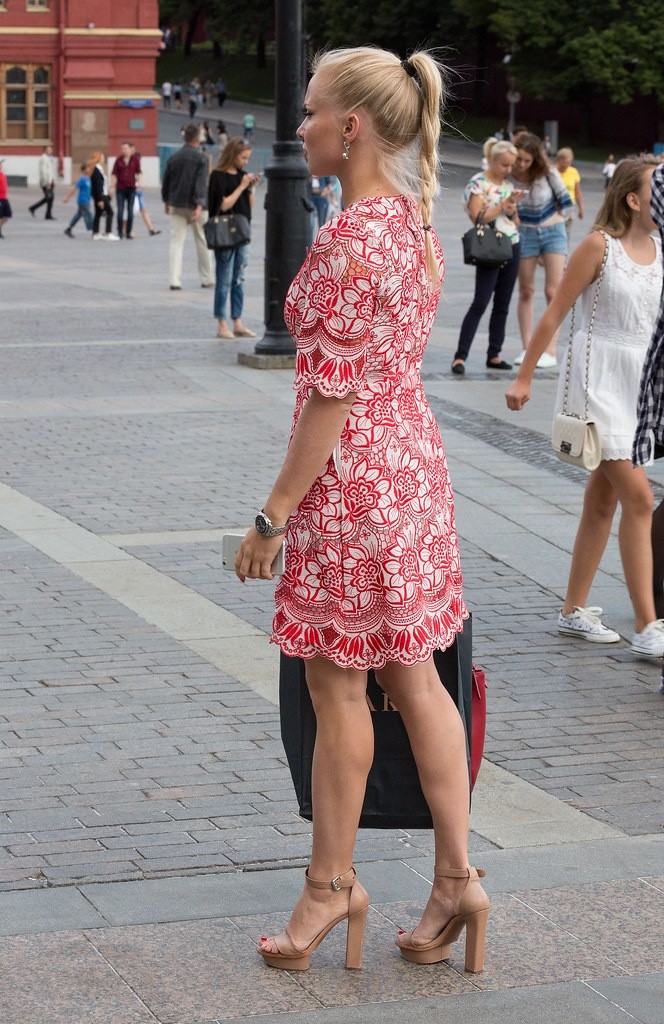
629 617 664 657
557 605 627 643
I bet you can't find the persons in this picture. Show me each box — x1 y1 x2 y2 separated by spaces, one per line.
0 159 12 238
553 148 585 268
162 77 227 120
309 175 342 229
505 155 664 658
235 46 488 971
199 110 257 155
451 132 572 374
207 137 259 338
29 146 57 219
162 125 215 289
63 141 162 241
162 26 179 52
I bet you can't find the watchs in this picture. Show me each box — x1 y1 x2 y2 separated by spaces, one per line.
255 508 290 537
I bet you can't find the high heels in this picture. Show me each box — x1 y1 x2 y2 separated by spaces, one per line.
255 863 369 970
395 865 489 974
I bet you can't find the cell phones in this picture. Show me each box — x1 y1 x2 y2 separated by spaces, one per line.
221 534 285 575
510 190 530 199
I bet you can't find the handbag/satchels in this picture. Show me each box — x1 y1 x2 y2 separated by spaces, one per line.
203 203 251 263
552 411 602 471
279 613 487 830
461 210 513 266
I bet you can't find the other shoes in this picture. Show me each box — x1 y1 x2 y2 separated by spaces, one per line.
200 277 216 288
234 328 256 337
64 225 162 241
451 360 465 374
486 358 512 370
171 281 183 290
513 350 528 364
536 352 558 367
45 215 54 220
29 207 34 217
217 331 236 339
